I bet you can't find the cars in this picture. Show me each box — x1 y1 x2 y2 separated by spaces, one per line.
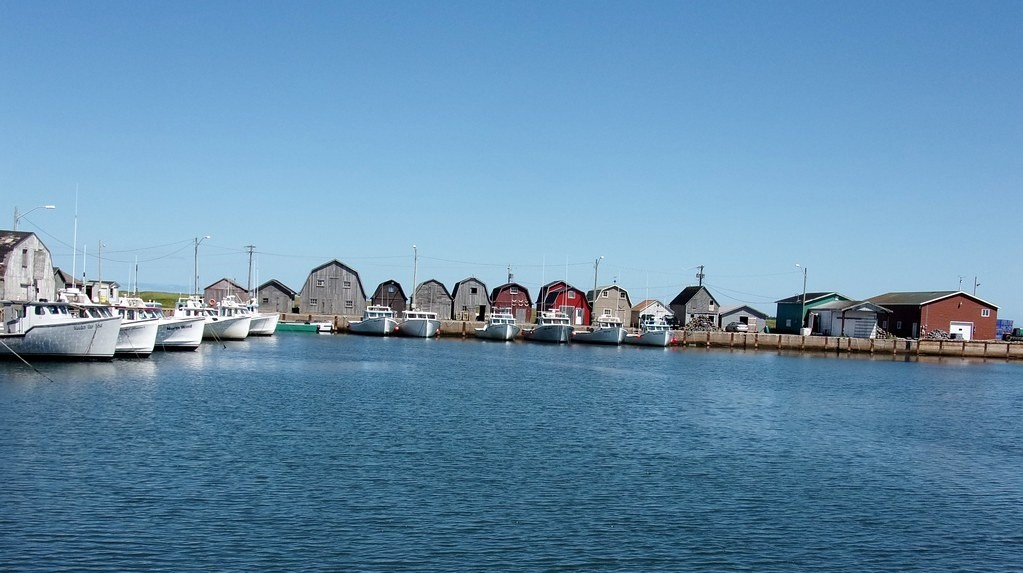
725 322 748 333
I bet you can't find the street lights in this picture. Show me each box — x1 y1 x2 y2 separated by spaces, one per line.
411 244 417 312
795 264 807 328
593 256 604 318
99 244 106 303
195 236 210 295
14 206 56 231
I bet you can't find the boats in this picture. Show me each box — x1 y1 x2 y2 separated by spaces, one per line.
474 307 520 341
398 310 441 337
0 300 123 362
347 304 399 336
522 309 574 341
571 314 628 342
626 317 673 346
55 288 334 358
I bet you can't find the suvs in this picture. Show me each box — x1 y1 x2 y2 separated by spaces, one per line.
637 314 655 328
660 315 680 330
